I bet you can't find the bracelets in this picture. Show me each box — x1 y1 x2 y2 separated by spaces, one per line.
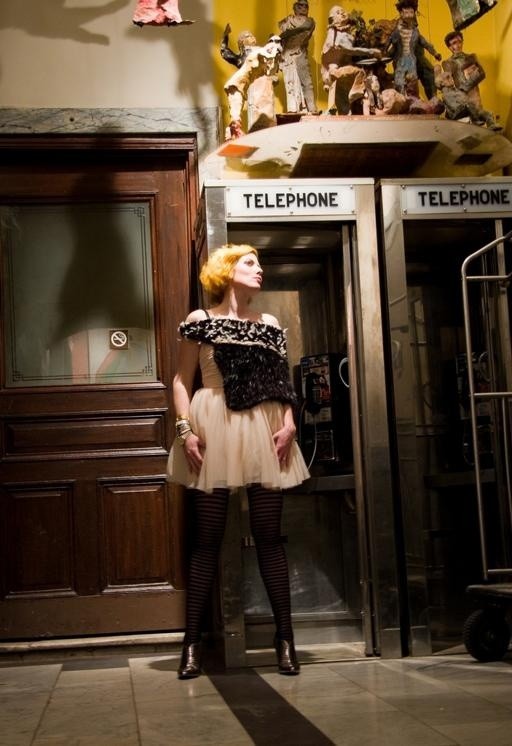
174 415 190 449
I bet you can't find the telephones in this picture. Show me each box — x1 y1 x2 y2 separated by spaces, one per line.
300 353 339 424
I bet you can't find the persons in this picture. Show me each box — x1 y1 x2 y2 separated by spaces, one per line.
166 243 311 680
222 2 502 138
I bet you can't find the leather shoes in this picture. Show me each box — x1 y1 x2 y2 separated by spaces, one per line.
273 632 300 675
177 636 203 677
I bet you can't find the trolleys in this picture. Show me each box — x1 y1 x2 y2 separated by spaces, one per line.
456 231 512 667
